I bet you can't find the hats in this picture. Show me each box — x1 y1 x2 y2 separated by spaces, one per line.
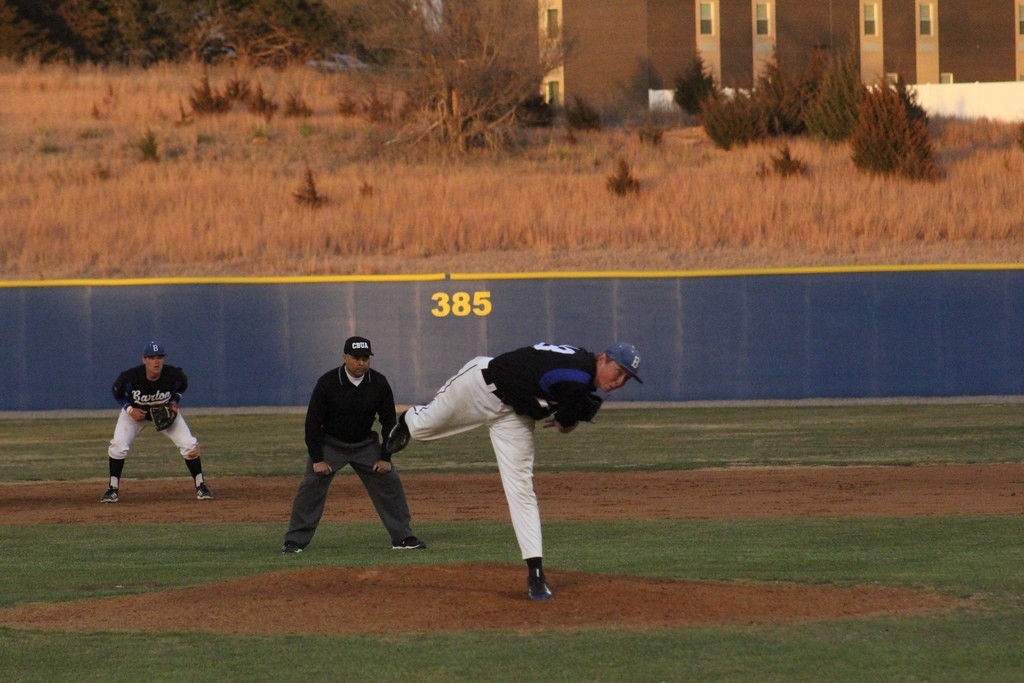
143 341 167 357
605 342 645 384
344 336 374 357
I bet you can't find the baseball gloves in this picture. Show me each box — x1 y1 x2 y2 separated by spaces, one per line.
150 401 179 432
578 392 612 425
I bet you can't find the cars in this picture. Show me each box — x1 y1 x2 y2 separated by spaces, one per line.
204 48 237 63
309 54 368 70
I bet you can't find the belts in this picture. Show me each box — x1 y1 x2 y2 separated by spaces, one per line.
482 369 511 406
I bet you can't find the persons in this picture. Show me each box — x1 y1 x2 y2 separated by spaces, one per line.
282 336 426 553
100 341 215 503
386 342 644 599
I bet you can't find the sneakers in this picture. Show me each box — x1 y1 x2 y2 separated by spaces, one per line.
196 482 214 500
392 536 426 550
100 486 119 503
527 568 554 600
281 543 303 554
386 410 411 453
